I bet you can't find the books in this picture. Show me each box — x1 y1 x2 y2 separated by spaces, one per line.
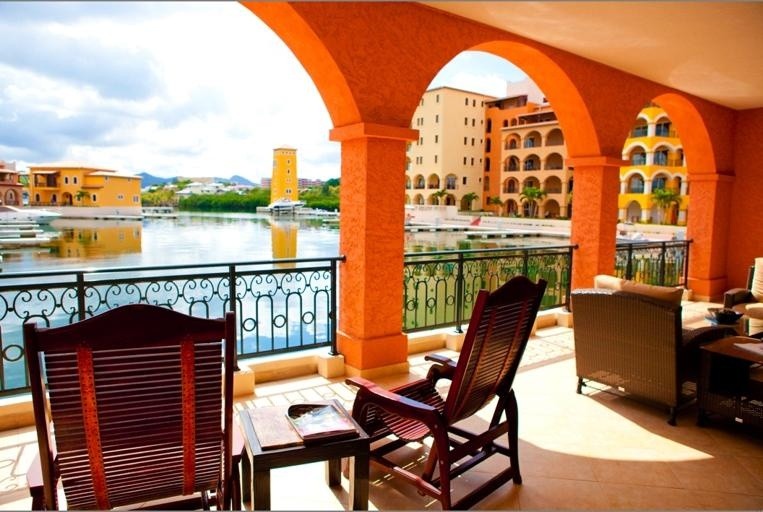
284 399 360 441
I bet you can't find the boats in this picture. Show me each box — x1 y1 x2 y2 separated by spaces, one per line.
1 204 62 225
145 206 180 221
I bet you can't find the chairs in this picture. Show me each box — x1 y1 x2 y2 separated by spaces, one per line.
24 304 244 510
570 274 738 427
341 276 547 511
722 256 763 337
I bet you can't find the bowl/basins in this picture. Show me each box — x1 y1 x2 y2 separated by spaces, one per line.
707 308 743 323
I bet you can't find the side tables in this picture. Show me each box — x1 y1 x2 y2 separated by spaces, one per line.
694 335 761 440
238 398 370 512
